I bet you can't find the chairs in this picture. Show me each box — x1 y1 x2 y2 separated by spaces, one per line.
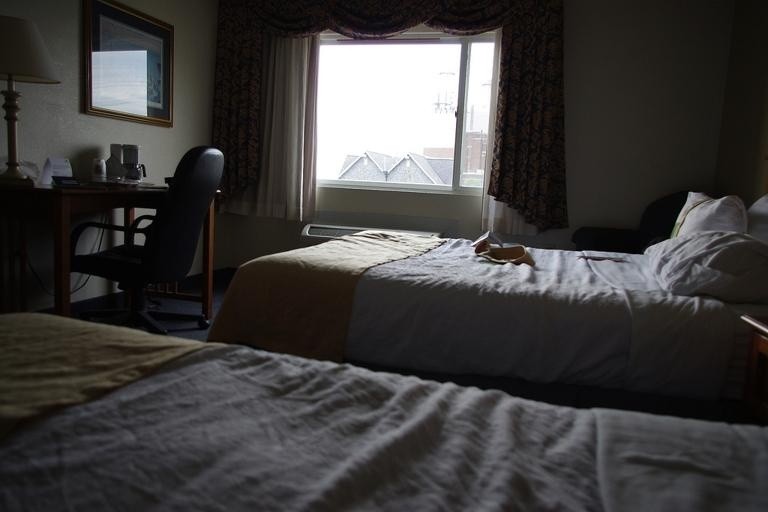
70 144 224 336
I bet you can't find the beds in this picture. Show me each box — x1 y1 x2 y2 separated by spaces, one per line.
1 312 768 511
206 228 766 402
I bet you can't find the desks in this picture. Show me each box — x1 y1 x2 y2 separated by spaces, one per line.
0 184 222 329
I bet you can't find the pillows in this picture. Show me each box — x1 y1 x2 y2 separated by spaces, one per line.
642 193 768 303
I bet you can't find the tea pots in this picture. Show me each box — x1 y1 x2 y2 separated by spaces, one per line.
121 144 146 180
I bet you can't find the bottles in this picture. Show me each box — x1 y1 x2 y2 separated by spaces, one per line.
91 158 106 182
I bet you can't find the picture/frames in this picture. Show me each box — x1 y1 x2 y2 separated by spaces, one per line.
80 2 174 128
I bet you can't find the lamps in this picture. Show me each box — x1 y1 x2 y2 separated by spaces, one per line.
0 14 61 188
